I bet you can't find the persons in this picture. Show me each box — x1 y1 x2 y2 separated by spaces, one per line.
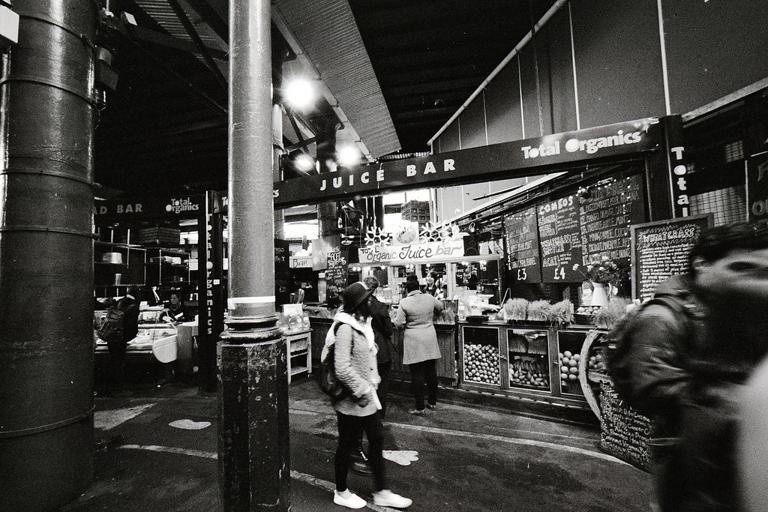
388 278 444 415
158 290 192 320
318 277 414 509
96 286 140 398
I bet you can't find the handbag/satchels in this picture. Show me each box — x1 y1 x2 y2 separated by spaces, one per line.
318 321 355 401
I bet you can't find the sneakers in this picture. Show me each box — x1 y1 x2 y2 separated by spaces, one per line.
333 488 367 510
371 489 413 508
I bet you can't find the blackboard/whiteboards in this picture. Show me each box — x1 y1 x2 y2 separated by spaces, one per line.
630 213 715 305
505 174 645 283
599 380 657 474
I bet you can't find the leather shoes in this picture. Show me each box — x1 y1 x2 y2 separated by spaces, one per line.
350 446 373 476
407 401 436 417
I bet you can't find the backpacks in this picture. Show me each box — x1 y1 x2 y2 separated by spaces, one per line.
97 299 136 342
602 297 707 412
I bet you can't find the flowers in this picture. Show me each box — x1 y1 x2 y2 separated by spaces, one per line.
573 253 618 283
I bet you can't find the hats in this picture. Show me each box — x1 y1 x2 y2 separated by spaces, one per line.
343 281 375 313
402 275 434 288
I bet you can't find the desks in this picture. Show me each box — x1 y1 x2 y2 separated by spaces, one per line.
279 328 314 386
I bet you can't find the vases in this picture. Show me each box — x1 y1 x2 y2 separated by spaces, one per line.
590 281 609 307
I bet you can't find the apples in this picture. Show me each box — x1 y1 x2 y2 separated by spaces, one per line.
464 343 500 385
